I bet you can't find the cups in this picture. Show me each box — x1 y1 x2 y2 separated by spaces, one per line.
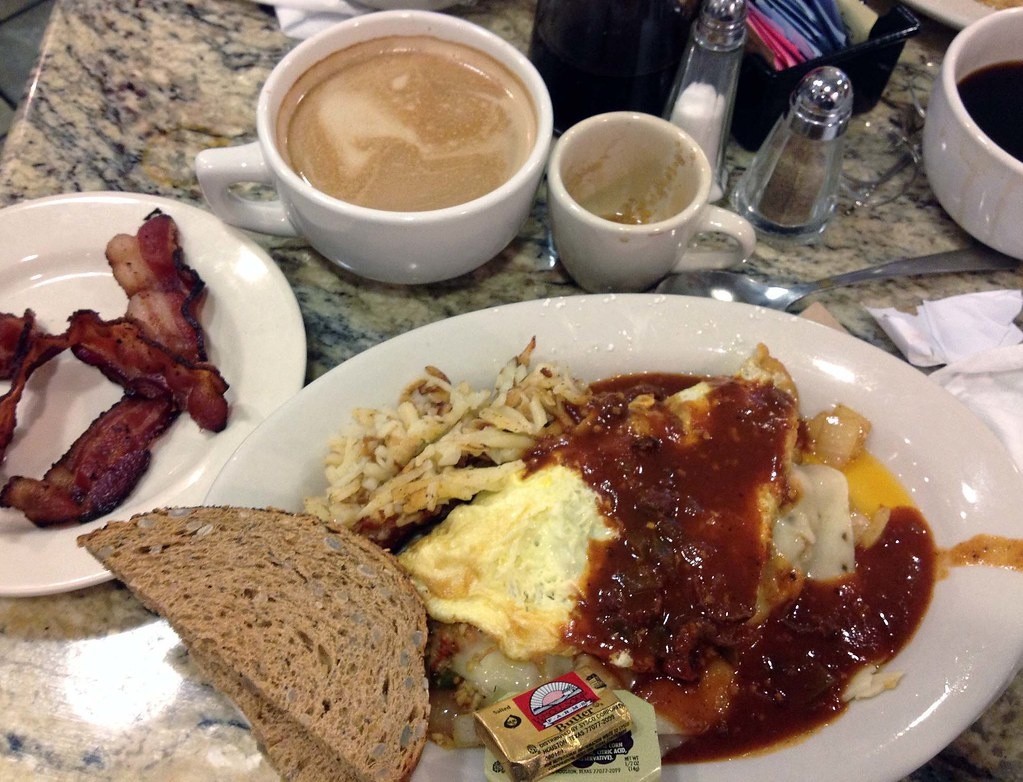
194 8 553 282
546 111 756 292
909 6 1023 259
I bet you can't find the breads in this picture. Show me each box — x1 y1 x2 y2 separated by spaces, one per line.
75 505 431 778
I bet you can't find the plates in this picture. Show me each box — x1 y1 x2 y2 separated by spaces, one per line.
196 294 1023 782
904 0 995 29
0 189 307 598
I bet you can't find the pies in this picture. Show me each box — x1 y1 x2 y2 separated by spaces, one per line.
394 346 802 688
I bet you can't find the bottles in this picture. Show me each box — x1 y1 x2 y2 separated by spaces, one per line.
729 66 854 245
663 0 750 203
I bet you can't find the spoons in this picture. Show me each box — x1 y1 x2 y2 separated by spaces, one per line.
654 243 1023 312
